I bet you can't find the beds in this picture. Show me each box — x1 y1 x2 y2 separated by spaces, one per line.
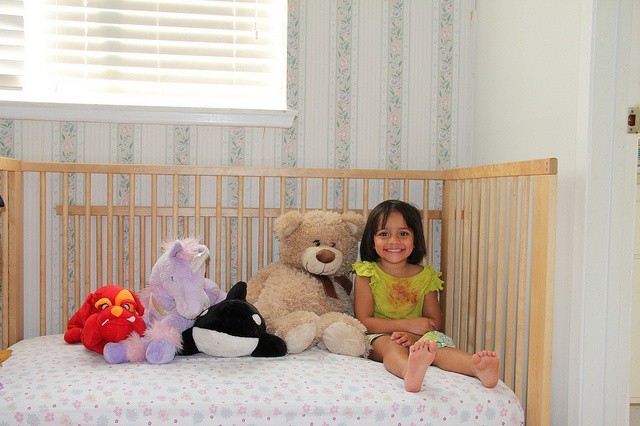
0 154 559 426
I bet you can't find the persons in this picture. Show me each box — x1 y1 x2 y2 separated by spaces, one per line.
352 199 500 393
